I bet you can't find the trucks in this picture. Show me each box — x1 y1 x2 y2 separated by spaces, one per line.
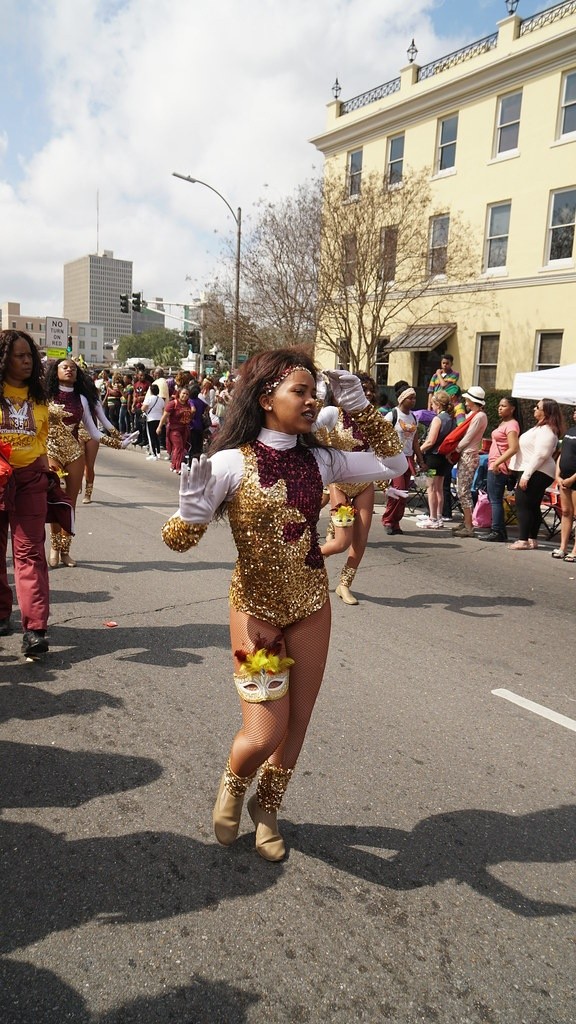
126 358 155 369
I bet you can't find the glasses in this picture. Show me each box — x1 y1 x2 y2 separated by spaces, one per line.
535 404 543 412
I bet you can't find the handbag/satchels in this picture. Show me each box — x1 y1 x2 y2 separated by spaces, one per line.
138 412 147 424
507 475 516 492
437 410 484 454
215 401 226 418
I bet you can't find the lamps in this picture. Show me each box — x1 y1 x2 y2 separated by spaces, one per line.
332 78 341 100
505 0 519 16
406 39 418 64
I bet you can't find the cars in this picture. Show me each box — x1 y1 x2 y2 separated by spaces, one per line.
237 354 247 363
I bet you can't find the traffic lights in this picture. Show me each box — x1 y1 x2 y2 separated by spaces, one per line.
120 294 128 313
186 330 200 354
131 291 142 312
67 336 72 353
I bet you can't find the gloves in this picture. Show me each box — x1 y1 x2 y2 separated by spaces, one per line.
122 431 138 449
323 370 370 414
385 487 410 499
177 455 217 522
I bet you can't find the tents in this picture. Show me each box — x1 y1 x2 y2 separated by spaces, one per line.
511 362 576 406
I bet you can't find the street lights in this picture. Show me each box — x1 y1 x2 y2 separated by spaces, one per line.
172 172 241 371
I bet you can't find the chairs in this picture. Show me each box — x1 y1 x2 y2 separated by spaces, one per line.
409 455 576 541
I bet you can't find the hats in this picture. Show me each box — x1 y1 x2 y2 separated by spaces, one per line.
460 387 485 405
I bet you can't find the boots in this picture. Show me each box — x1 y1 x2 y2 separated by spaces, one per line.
48 532 60 568
335 562 358 605
59 530 77 566
214 754 257 846
247 760 295 862
83 481 94 504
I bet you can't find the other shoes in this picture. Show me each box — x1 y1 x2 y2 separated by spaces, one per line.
20 628 49 656
416 513 576 562
384 526 394 534
393 528 403 534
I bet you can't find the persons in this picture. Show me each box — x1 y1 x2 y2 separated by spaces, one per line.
44 357 140 567
160 350 408 858
76 361 140 508
0 329 67 653
95 355 576 561
